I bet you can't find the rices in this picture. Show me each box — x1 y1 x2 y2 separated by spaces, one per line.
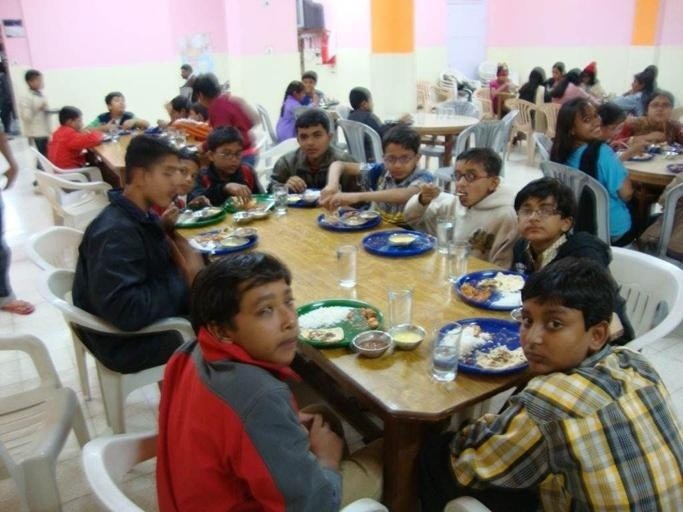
490 293 525 306
297 306 353 328
441 325 491 356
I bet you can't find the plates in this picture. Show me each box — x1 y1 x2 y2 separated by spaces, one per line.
621 135 683 175
175 207 226 228
189 227 259 256
225 194 277 213
287 192 319 209
316 208 381 233
294 298 385 349
455 268 528 311
94 124 205 154
431 317 529 373
361 229 435 258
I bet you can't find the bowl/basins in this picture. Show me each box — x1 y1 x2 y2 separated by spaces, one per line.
248 207 271 219
351 329 392 359
234 211 253 225
388 324 427 350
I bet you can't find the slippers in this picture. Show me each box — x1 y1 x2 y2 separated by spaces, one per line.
1 298 35 317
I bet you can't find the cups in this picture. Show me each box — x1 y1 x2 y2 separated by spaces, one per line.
387 289 414 329
274 184 290 215
435 214 456 254
430 322 464 380
336 244 358 288
446 239 471 284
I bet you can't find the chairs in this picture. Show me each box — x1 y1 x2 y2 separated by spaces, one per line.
338 496 492 512
406 76 683 350
21 137 115 269
0 268 197 512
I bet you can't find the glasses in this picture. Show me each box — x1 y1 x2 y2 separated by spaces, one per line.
452 172 488 183
516 207 568 218
211 148 243 160
